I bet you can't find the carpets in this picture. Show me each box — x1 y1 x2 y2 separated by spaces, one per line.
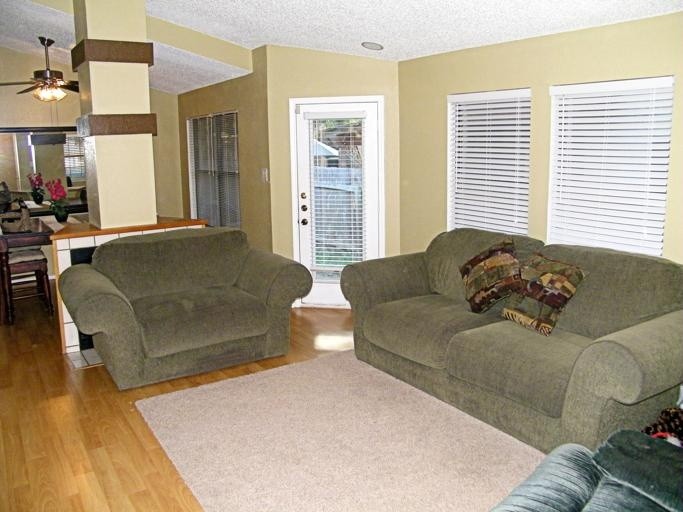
135 347 548 512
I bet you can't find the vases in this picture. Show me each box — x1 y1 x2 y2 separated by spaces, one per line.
33 193 43 204
53 206 68 223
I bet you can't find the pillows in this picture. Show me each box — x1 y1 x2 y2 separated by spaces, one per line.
499 251 589 338
458 235 522 312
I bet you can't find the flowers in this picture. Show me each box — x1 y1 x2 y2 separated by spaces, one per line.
45 179 71 215
27 171 45 197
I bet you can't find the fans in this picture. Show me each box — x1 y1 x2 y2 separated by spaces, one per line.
0 36 80 94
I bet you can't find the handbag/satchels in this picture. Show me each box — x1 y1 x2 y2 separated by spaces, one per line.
0 198 31 234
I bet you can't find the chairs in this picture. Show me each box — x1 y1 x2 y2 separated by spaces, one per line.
58 225 314 389
0 232 56 322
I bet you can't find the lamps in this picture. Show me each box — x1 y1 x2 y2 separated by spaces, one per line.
33 84 67 102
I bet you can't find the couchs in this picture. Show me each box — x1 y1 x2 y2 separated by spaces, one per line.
339 227 683 459
485 429 683 512
